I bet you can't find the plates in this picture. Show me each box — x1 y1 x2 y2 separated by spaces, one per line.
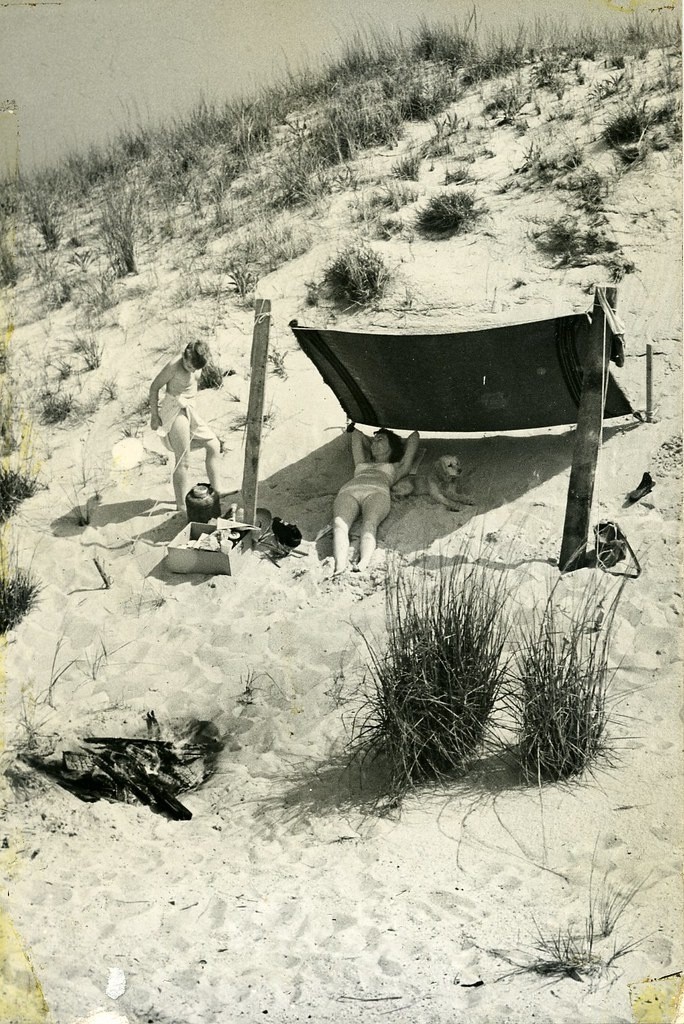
255 508 271 535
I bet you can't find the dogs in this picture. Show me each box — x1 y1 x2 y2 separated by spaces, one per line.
391 454 475 511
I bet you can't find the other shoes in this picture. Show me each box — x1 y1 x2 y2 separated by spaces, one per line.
631 472 656 500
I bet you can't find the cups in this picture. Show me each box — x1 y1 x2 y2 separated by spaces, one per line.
220 540 233 555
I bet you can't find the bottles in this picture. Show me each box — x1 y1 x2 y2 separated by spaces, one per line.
186 483 221 523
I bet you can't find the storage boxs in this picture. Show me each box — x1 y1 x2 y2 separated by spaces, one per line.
167 522 252 576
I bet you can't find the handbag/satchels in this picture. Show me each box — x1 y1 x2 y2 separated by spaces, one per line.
594 521 642 579
272 516 303 548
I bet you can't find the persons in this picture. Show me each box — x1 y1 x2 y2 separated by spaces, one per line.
149 339 225 511
332 426 420 576
